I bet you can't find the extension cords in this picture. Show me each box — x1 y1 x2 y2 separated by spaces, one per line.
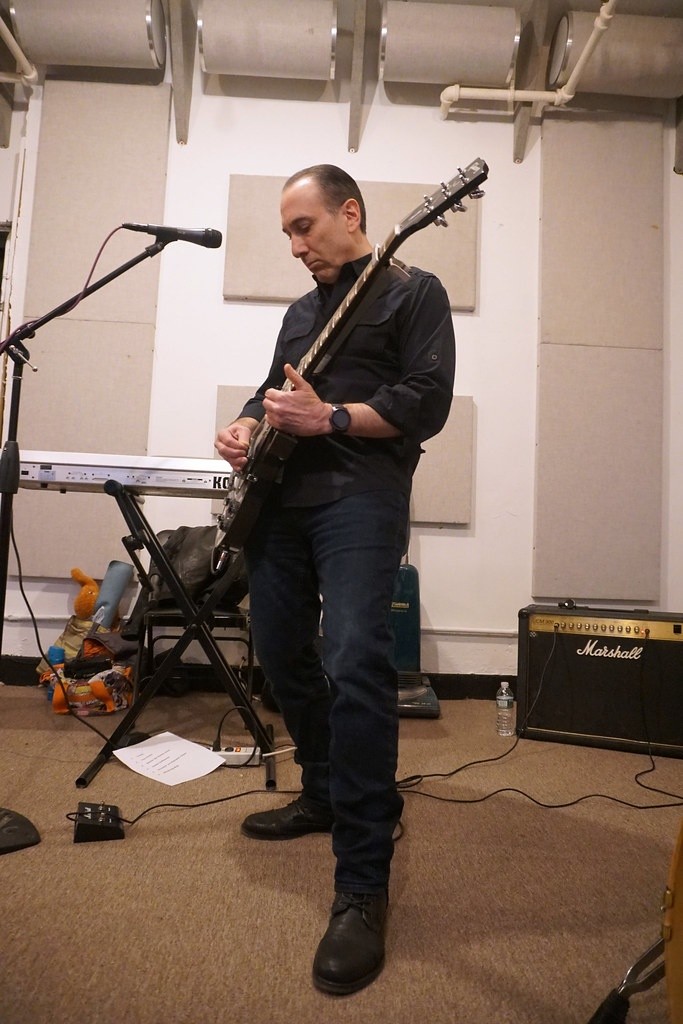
205 746 261 765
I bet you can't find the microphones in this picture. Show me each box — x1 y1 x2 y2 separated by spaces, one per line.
122 223 222 248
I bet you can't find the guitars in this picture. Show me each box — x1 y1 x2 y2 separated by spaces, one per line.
213 154 492 558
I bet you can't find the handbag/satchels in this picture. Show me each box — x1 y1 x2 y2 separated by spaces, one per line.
35 617 148 715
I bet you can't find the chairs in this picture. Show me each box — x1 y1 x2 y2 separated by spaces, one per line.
126 529 256 710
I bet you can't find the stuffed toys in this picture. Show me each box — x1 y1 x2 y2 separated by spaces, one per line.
39 567 146 716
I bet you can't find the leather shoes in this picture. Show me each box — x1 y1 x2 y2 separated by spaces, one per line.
241 799 336 841
312 887 388 995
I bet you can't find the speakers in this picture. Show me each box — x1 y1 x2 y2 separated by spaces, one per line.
514 604 683 759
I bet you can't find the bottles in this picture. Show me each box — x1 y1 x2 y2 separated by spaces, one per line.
496 681 514 737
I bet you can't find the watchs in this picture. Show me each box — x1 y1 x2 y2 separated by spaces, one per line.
327 401 353 433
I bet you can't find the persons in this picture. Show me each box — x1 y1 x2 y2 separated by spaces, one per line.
213 163 455 995
